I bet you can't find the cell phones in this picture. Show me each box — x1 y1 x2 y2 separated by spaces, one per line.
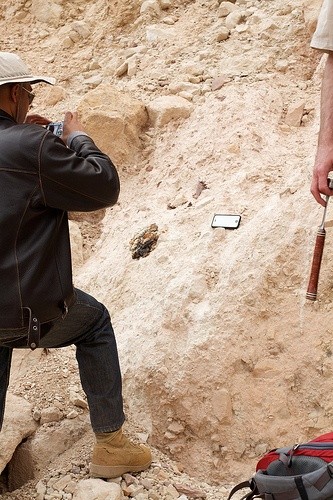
211 214 241 228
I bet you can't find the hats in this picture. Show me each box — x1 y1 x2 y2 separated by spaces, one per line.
0 52 56 87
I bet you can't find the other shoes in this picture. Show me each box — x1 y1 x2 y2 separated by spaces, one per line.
90 425 153 477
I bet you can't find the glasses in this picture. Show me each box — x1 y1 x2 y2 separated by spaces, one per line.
22 87 35 105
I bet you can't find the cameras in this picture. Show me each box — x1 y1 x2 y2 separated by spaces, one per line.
45 122 64 137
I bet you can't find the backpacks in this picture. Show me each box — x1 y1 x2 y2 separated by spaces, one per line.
227 431 333 500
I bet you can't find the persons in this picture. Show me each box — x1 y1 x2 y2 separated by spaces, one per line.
306 0 333 208
0 49 153 479
